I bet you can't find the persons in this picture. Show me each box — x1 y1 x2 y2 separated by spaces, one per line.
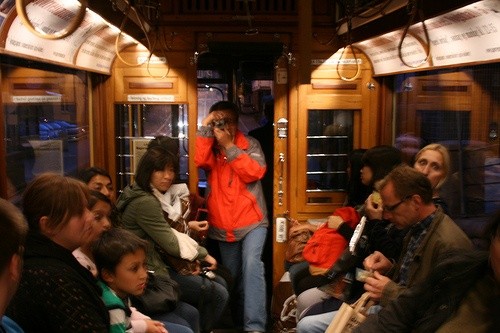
289 143 500 333
0 137 230 333
193 100 270 333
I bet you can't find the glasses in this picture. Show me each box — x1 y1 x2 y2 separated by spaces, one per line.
382 193 422 211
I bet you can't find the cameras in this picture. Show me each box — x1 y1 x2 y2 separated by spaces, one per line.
212 119 225 130
200 261 212 278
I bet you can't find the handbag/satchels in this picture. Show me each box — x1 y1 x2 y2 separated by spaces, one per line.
284 216 316 264
325 292 378 333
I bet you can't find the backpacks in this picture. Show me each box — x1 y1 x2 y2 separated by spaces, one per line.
301 206 360 269
